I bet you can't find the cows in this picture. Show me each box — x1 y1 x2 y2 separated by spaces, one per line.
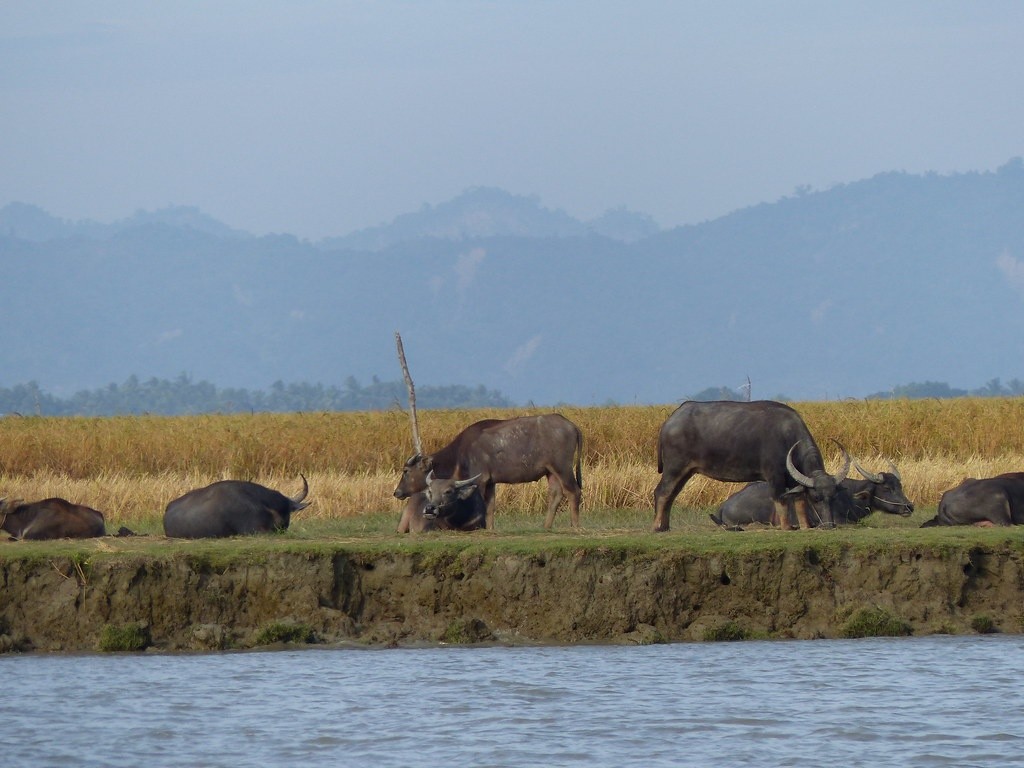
938 472 1024 526
163 472 313 539
0 497 106 541
393 412 584 534
653 399 915 532
